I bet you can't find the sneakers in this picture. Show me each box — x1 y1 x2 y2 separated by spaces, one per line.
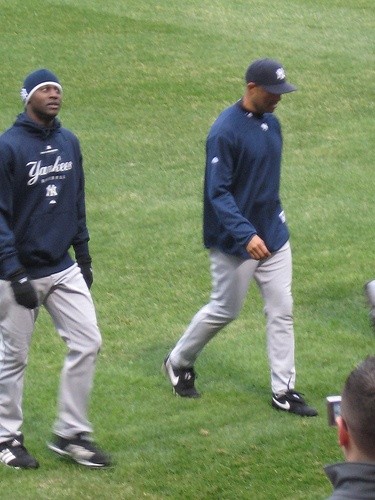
0 434 40 470
47 437 107 467
272 390 318 416
164 353 199 396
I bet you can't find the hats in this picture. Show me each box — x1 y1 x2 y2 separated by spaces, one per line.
245 58 296 94
21 69 62 107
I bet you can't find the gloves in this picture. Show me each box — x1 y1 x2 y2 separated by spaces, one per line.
11 275 39 309
81 267 93 289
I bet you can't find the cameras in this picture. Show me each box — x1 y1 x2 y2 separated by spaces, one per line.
326 395 343 426
